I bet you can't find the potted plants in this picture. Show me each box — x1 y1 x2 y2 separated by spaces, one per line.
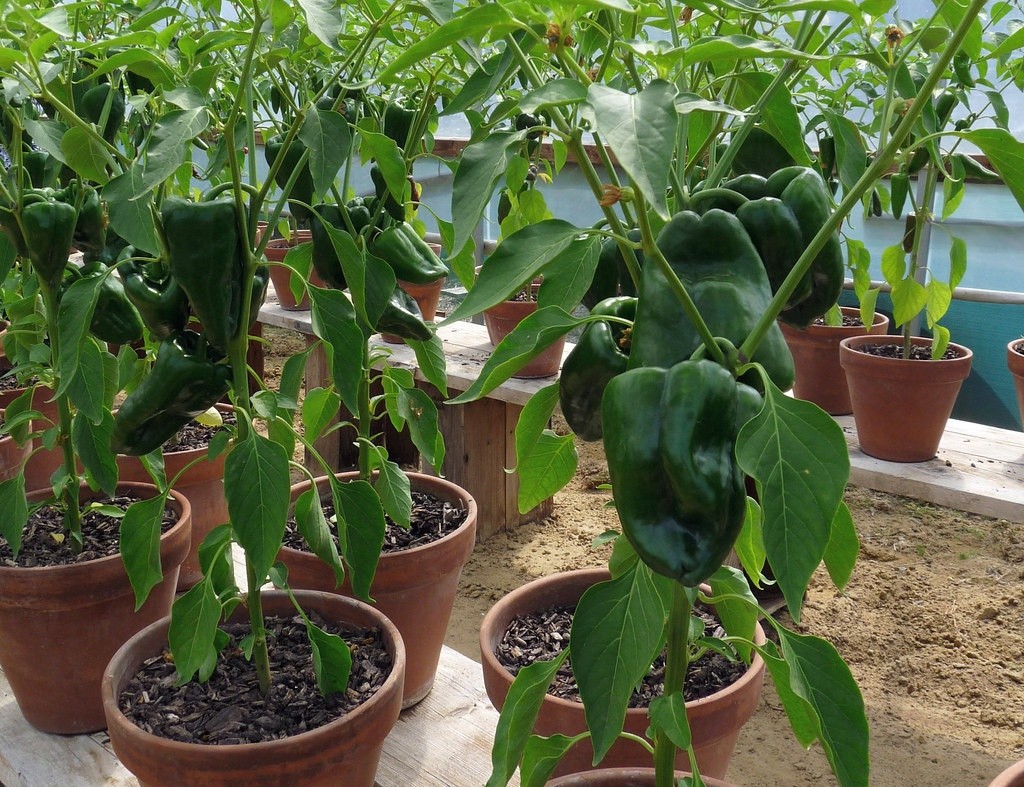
0 0 1024 787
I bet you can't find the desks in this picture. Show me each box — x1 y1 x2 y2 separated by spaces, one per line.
61 241 1024 539
0 539 521 787
